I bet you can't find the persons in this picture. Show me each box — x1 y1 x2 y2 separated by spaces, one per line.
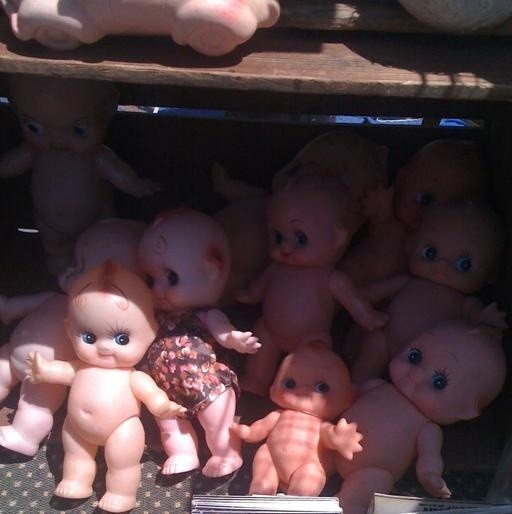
0 0 512 514
0 1 512 514
